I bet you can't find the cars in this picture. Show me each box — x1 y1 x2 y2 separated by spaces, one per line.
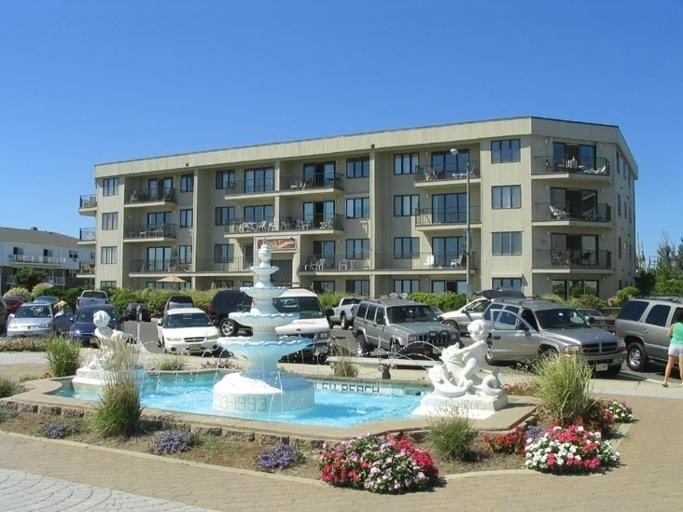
571 306 616 334
120 301 153 321
0 296 118 347
154 307 220 355
433 295 490 339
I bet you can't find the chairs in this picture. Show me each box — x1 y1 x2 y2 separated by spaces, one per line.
79 195 96 275
546 154 607 269
423 253 463 270
128 189 177 272
421 160 475 181
236 173 350 271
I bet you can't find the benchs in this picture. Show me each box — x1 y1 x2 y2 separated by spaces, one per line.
324 353 441 380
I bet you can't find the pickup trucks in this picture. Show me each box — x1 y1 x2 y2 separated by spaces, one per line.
74 288 110 311
325 295 359 331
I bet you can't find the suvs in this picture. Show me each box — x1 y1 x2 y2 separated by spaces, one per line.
478 288 628 380
349 297 463 360
614 296 683 372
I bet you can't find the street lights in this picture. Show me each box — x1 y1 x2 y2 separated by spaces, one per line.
445 147 472 299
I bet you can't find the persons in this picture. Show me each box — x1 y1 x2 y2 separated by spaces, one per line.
663 308 683 387
448 320 492 386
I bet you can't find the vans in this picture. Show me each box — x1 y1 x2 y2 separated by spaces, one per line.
162 294 194 317
271 288 331 358
207 287 335 344
